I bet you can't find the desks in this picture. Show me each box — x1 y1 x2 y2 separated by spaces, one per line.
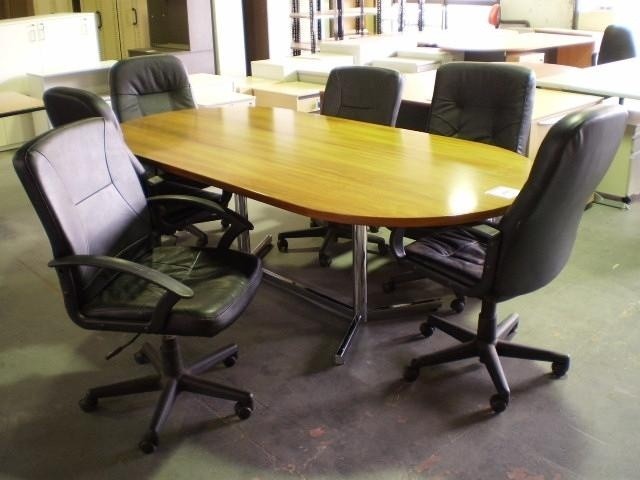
1 12 255 155
122 105 535 364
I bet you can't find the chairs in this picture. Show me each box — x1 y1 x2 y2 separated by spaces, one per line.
108 54 233 247
382 62 535 311
402 101 627 413
12 117 263 455
275 66 403 266
42 87 233 244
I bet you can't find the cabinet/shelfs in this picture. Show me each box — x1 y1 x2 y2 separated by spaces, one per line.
79 1 150 63
